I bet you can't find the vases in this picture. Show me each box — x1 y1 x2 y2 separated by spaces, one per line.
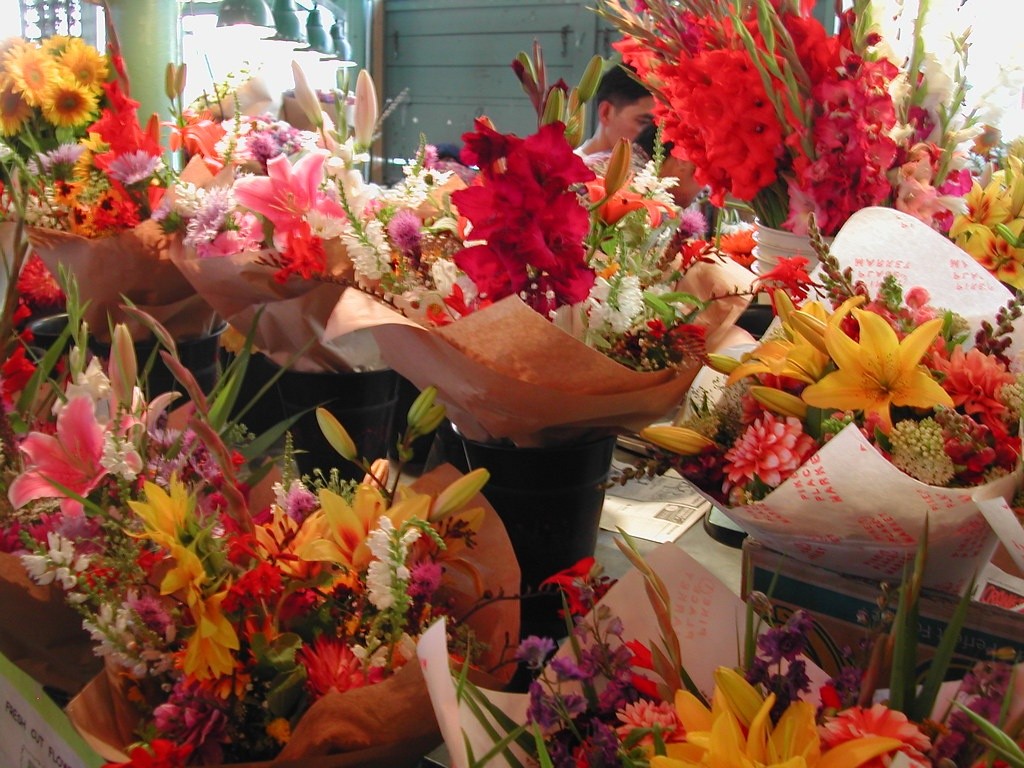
262 354 399 486
26 311 230 414
439 420 616 693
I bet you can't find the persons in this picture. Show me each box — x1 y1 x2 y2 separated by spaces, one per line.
571 60 656 160
582 122 707 226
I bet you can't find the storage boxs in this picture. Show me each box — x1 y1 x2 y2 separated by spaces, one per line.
740 532 1024 691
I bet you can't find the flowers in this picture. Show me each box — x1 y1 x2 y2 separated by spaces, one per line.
13 387 521 768
417 509 1024 768
0 32 109 167
588 0 1024 302
0 0 222 336
136 56 427 374
336 41 752 443
637 206 1024 508
0 262 329 597
